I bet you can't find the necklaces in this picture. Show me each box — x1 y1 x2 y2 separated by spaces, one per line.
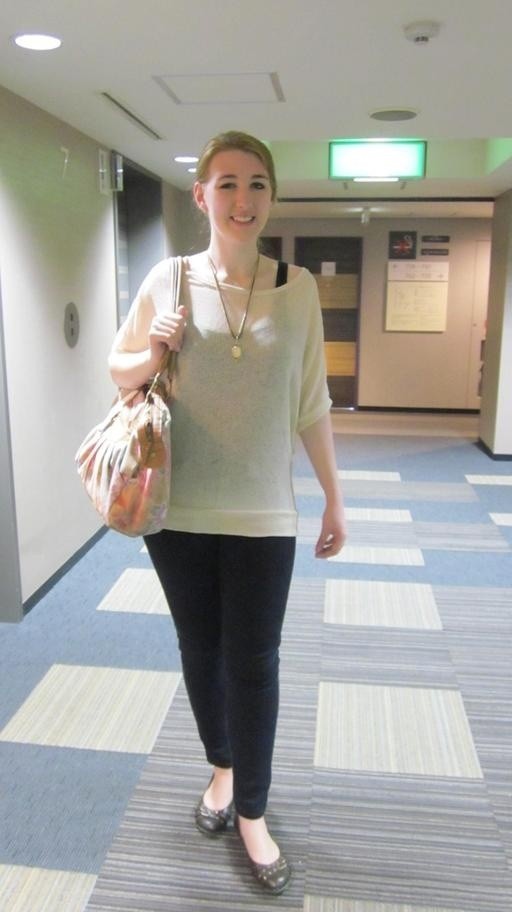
202 248 262 360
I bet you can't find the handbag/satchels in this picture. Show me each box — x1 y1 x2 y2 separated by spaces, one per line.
75 377 171 537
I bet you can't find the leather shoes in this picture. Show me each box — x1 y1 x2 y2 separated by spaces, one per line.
232 811 292 895
195 773 234 836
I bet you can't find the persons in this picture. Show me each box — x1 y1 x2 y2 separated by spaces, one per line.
108 130 350 895
